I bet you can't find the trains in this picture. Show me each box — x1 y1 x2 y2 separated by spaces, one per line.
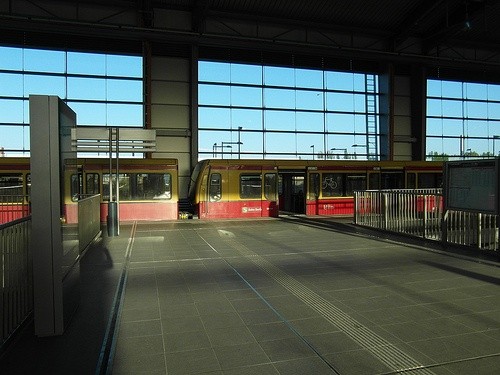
0 156 447 224
